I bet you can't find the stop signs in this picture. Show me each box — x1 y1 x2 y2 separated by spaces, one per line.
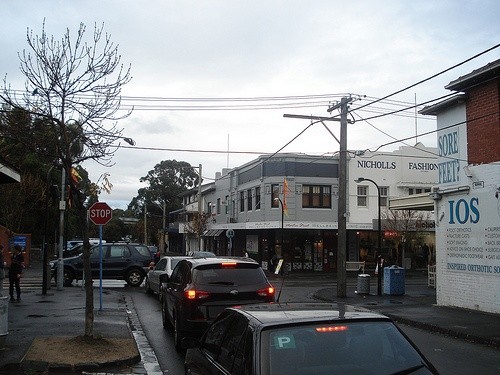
89 202 113 225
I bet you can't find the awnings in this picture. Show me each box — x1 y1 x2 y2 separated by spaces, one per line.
204 229 223 236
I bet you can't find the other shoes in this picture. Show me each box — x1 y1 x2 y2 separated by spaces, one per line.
10 296 15 301
17 296 21 300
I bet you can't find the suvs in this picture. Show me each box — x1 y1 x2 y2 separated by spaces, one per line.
48 242 155 288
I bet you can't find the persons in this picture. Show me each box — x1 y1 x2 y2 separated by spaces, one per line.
0 245 7 297
8 245 24 303
164 242 168 253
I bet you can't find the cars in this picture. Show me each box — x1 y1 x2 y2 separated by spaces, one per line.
188 251 216 258
145 253 195 294
54 237 106 259
159 256 277 340
177 302 438 375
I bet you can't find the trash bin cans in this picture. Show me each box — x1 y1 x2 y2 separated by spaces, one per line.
0 296 9 337
383 265 406 296
357 273 371 294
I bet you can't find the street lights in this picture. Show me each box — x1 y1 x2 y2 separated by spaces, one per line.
353 177 382 298
56 131 136 291
274 198 283 276
